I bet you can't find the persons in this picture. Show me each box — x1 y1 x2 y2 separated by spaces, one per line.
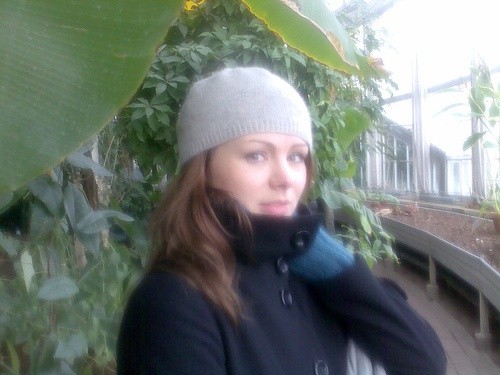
116 66 448 375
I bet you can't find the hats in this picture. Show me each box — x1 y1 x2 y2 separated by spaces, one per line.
173 66 312 167
